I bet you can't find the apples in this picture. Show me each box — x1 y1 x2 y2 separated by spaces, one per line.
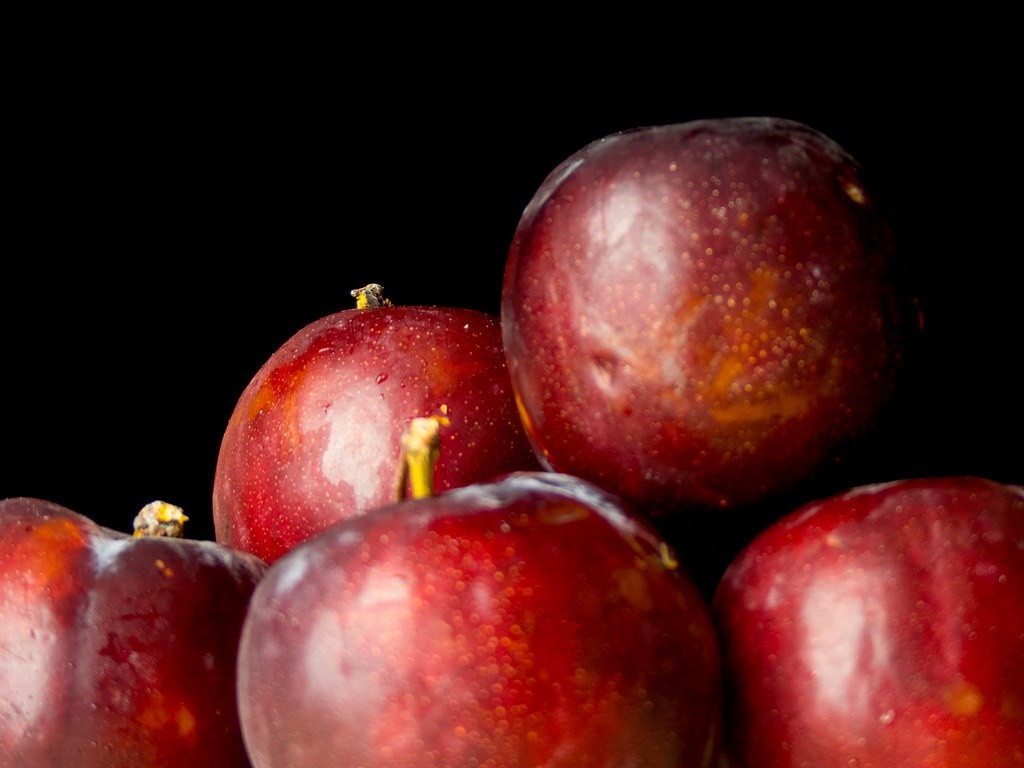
210 283 546 563
503 113 915 538
0 495 274 768
705 474 1024 768
233 416 727 768
